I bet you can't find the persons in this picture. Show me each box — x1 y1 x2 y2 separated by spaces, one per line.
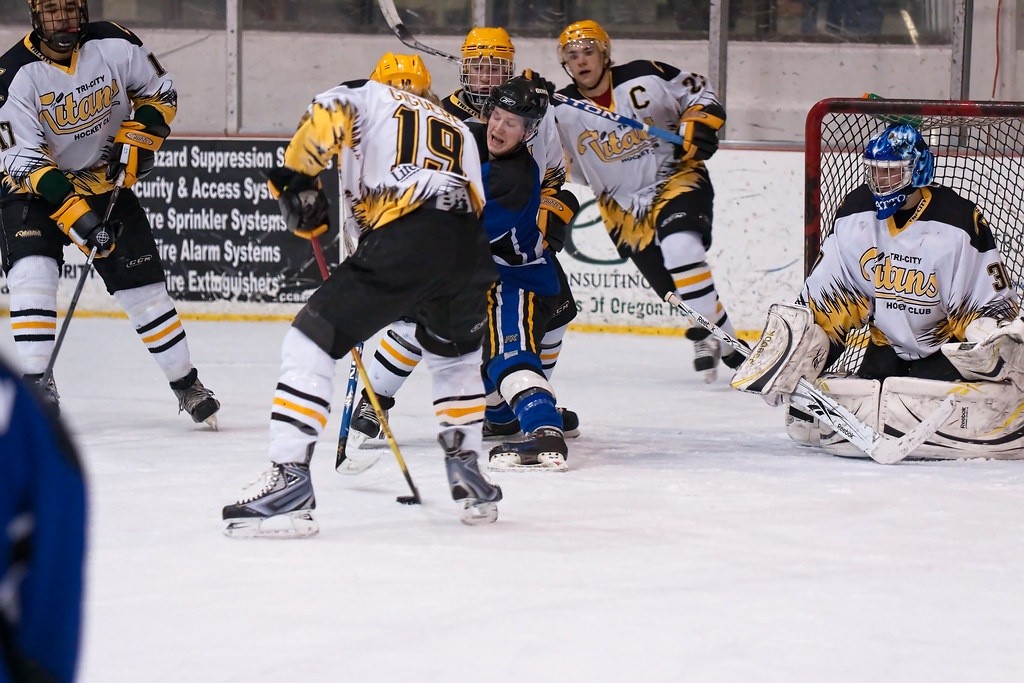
222 27 581 521
0 0 221 424
729 123 1024 461
521 19 752 372
0 352 91 683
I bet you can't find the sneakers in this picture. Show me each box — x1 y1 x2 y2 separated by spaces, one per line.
445 451 503 527
347 387 395 449
485 428 570 473
222 462 319 541
482 406 581 442
169 368 221 434
684 327 721 384
27 372 61 418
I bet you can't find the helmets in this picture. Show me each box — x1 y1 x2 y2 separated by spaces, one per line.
557 19 612 61
462 26 515 67
370 50 431 98
862 121 935 221
482 75 550 132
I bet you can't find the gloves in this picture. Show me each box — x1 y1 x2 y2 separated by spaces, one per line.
673 104 727 164
537 189 581 254
49 193 125 259
266 166 330 239
523 68 556 100
104 120 170 188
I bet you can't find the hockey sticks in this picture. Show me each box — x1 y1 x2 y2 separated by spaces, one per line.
35 169 128 399
309 236 423 507
335 343 384 478
379 2 684 145
667 289 957 465
